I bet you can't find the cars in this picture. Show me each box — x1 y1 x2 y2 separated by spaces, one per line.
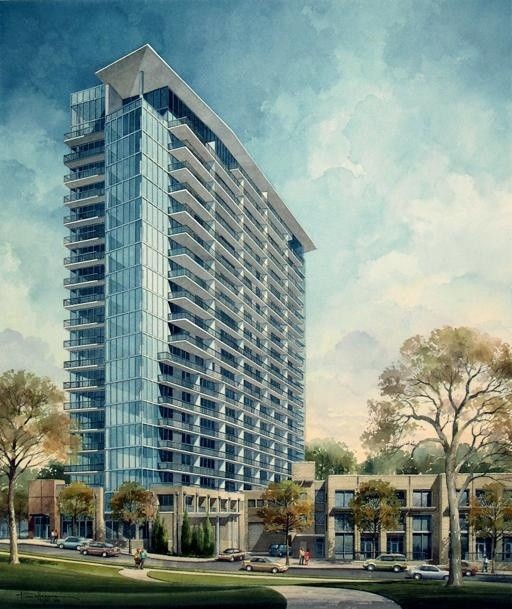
218 548 246 562
78 541 121 558
408 564 450 581
461 560 479 576
242 556 289 573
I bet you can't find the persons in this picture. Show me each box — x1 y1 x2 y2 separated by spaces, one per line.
135 548 147 569
482 556 489 572
51 529 58 544
300 547 310 566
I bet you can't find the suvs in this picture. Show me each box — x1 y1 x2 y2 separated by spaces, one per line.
268 543 293 557
363 553 408 573
55 535 86 551
